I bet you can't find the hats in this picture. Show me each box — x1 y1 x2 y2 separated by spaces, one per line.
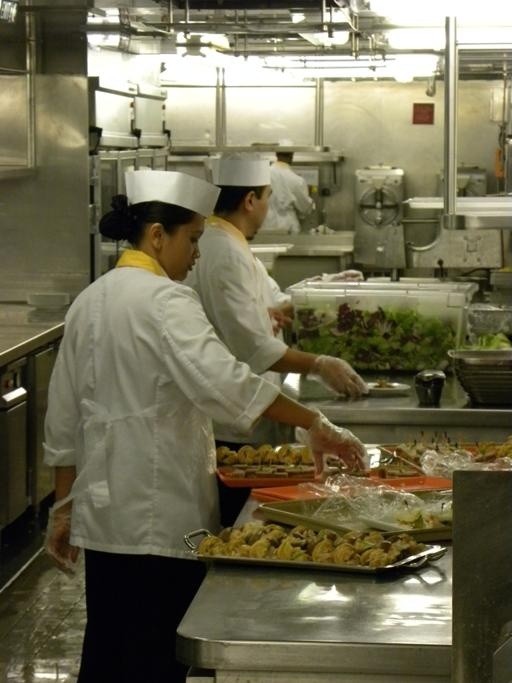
123 169 222 220
204 155 273 187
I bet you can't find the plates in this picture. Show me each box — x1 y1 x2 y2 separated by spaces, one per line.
363 380 411 396
357 510 406 532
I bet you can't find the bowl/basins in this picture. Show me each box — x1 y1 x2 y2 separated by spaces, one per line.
463 305 512 334
447 346 512 406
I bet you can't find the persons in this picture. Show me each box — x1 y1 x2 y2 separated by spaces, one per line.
45 168 366 682
258 137 318 234
175 154 371 527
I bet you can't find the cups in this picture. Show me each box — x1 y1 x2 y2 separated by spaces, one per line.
413 371 445 403
